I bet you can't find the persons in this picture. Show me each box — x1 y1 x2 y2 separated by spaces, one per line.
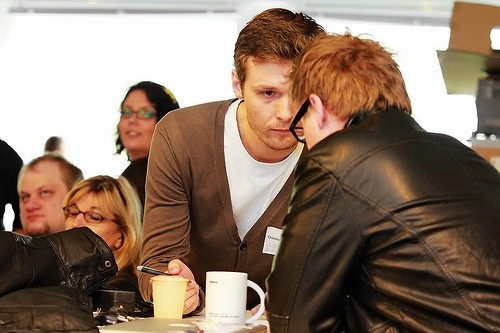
138 8 326 320
115 81 180 229
16 137 84 238
62 174 142 278
264 34 500 333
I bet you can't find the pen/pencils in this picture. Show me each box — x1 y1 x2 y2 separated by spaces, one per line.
137 266 194 283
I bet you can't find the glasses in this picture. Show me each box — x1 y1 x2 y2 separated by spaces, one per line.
289 99 310 143
120 108 156 120
63 206 120 224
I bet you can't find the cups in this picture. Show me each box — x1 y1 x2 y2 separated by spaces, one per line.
205 271 265 328
150 276 191 319
